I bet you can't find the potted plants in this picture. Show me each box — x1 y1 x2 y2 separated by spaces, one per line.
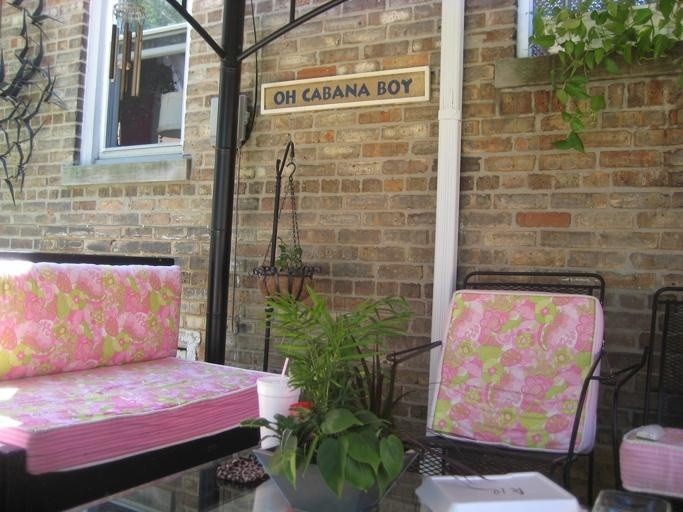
541 0 683 152
253 237 322 304
237 281 425 512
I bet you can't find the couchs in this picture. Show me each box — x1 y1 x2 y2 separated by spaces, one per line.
0 249 262 506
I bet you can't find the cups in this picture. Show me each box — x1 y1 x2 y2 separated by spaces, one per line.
256 376 302 452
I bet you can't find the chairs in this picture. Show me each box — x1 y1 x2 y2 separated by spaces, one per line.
384 267 604 495
606 282 681 511
